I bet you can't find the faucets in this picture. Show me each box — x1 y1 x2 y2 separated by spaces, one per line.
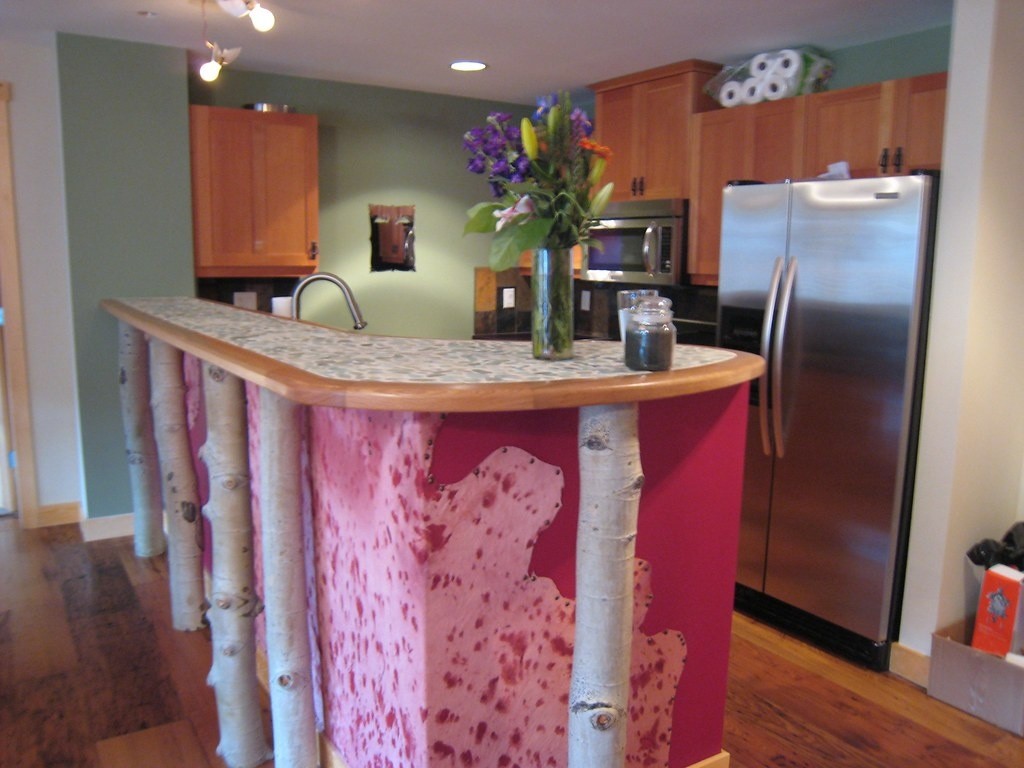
290 271 365 329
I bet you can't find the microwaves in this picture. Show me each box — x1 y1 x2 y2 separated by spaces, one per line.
579 215 684 286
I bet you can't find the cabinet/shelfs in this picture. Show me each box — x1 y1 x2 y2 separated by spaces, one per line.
520 58 949 287
188 105 320 278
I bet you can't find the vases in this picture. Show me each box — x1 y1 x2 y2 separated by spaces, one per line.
530 248 573 361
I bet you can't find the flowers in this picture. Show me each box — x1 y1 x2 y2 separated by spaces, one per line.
463 87 616 351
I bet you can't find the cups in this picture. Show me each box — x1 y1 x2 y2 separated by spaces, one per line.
616 289 658 343
234 292 257 309
271 296 295 318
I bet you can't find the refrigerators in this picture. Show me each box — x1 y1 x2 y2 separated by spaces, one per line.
712 171 935 645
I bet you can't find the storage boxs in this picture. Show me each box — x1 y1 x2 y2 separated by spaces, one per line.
927 558 1024 736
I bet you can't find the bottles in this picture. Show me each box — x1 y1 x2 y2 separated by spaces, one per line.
623 298 677 371
